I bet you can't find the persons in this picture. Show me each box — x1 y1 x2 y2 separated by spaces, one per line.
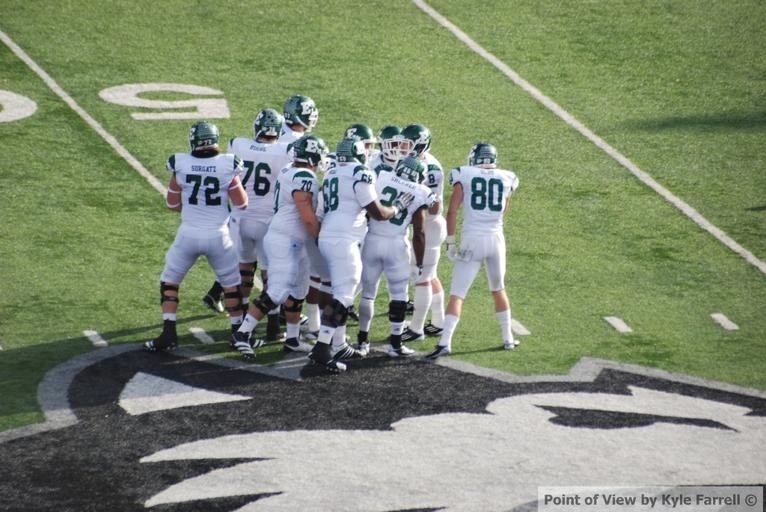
143 92 523 369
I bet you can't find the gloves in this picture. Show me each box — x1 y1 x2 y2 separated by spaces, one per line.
395 192 415 212
447 244 458 263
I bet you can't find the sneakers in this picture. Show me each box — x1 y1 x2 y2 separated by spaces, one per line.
141 338 177 352
202 290 452 374
504 338 520 350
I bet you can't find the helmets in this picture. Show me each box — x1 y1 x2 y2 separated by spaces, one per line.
188 120 220 151
469 143 497 166
253 95 432 184
